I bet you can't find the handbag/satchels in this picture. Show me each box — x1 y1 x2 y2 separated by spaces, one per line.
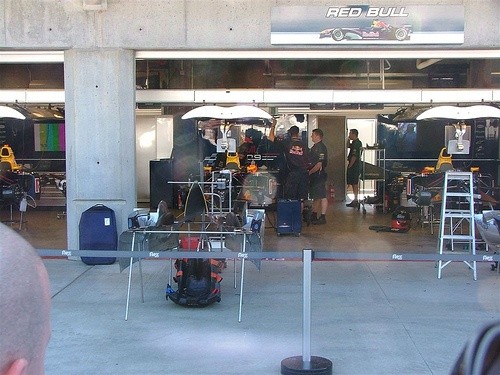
79 204 118 265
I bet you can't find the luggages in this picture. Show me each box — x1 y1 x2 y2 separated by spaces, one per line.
276 198 302 237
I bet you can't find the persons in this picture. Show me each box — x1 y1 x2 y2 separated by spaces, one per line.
238 128 262 168
0 222 52 375
305 129 329 225
346 129 362 208
401 122 416 152
267 117 312 220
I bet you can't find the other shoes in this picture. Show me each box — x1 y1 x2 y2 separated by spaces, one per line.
304 215 326 224
346 202 359 207
